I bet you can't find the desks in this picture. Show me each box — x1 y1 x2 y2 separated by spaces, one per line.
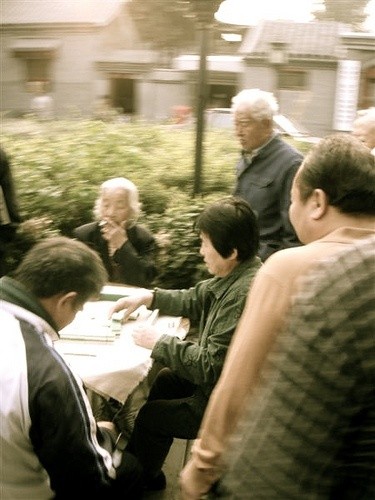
58 284 191 420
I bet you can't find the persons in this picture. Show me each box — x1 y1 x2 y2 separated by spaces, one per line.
0 236 144 500
178 107 375 500
231 87 306 262
109 199 265 491
73 176 160 287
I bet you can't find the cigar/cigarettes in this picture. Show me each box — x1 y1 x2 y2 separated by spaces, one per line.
97 220 109 226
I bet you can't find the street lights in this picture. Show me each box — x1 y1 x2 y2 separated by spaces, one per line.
190 0 223 201
